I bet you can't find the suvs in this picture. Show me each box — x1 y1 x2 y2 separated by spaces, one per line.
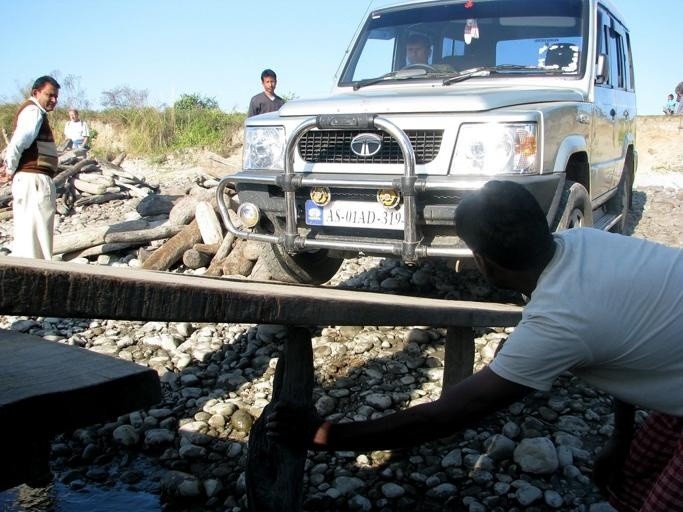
216 0 637 283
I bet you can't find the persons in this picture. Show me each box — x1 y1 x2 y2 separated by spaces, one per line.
675 81 683 115
401 35 456 73
264 182 683 512
4 75 61 261
65 110 89 148
663 95 677 115
248 69 286 119
